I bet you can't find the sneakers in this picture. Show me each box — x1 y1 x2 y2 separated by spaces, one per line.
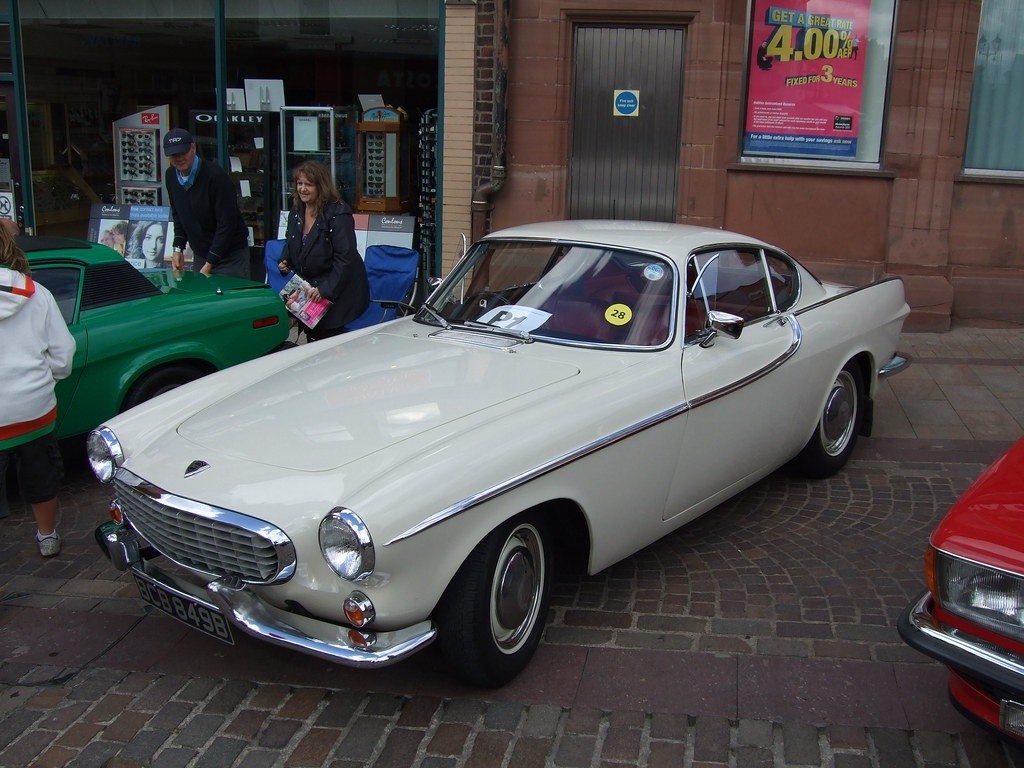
33 532 61 556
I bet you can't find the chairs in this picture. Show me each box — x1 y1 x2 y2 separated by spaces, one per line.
343 244 421 331
611 265 705 346
264 237 294 294
537 258 627 345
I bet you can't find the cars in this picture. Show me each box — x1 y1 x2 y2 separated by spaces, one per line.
83 217 916 689
899 430 1024 743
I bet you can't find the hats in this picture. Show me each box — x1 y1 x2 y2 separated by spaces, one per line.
163 128 195 157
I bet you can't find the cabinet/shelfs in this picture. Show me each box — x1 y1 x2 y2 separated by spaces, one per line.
280 104 337 211
355 104 413 213
189 108 272 246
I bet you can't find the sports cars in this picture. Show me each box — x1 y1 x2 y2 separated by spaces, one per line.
0 235 290 439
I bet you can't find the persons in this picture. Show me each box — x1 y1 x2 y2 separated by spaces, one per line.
282 288 311 313
100 230 115 248
129 220 167 270
276 159 370 344
112 221 131 257
163 128 252 281
1 215 78 559
